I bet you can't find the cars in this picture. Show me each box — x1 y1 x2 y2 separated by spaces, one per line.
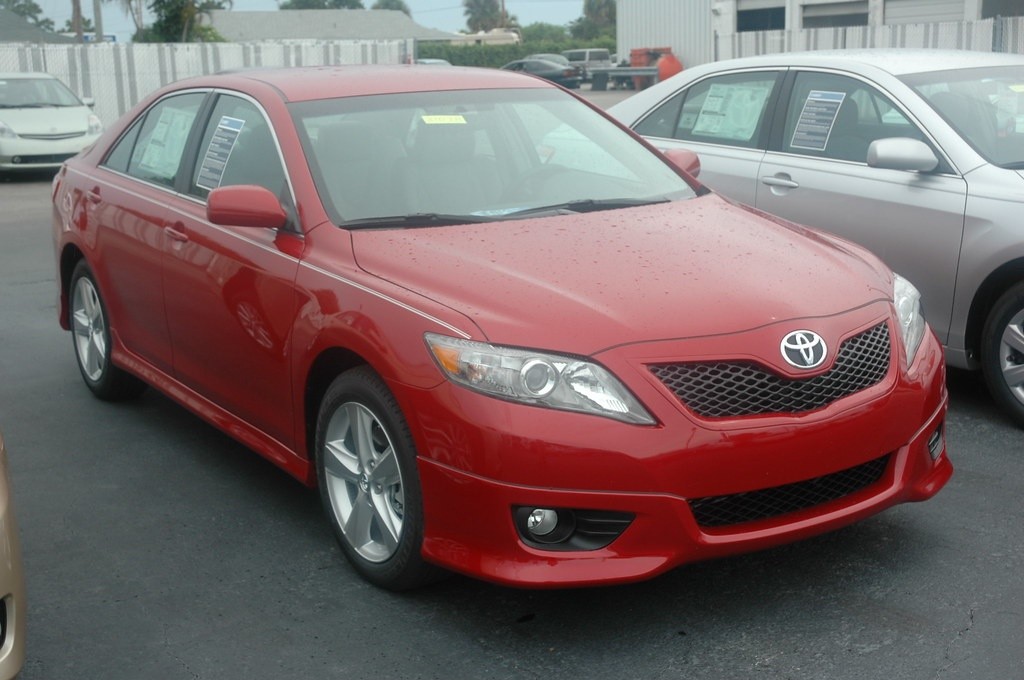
48 64 953 596
0 72 104 182
538 48 1024 425
414 58 451 66
497 59 581 90
610 54 617 66
523 53 570 67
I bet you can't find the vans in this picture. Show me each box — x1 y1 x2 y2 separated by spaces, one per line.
561 48 613 83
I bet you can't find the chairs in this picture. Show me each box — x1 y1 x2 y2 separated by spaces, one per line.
221 111 497 230
820 90 997 170
